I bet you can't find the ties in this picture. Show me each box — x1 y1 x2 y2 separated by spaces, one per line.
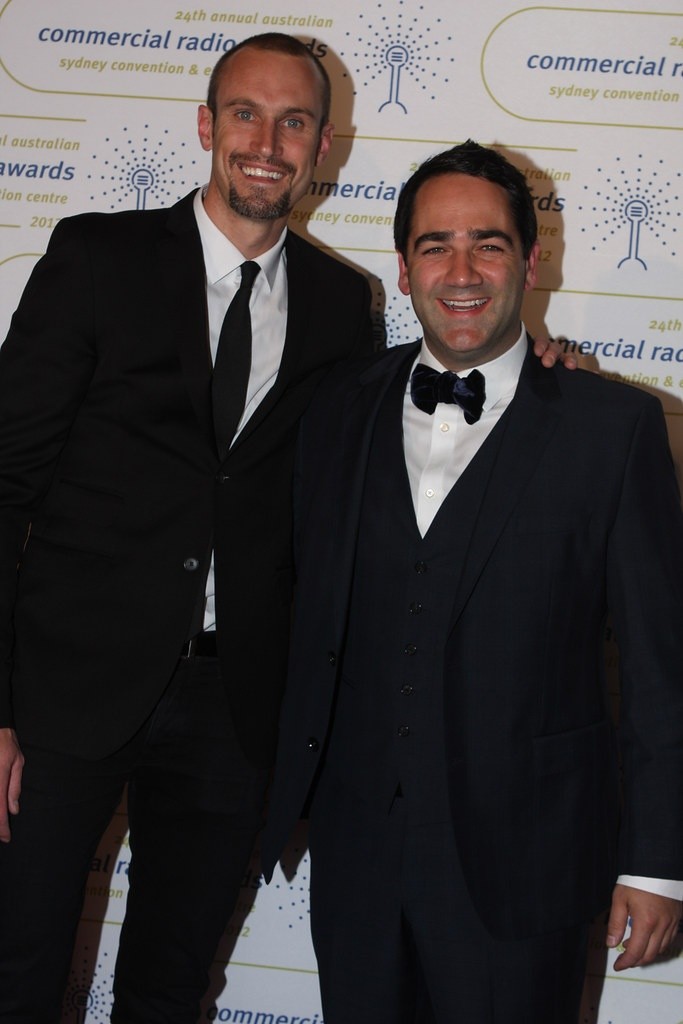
211 260 262 467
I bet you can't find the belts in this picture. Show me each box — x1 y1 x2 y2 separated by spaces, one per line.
180 634 219 658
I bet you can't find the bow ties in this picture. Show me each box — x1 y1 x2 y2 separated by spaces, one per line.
410 362 486 425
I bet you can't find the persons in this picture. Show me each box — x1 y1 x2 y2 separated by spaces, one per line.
246 141 683 1024
1 32 600 1024
